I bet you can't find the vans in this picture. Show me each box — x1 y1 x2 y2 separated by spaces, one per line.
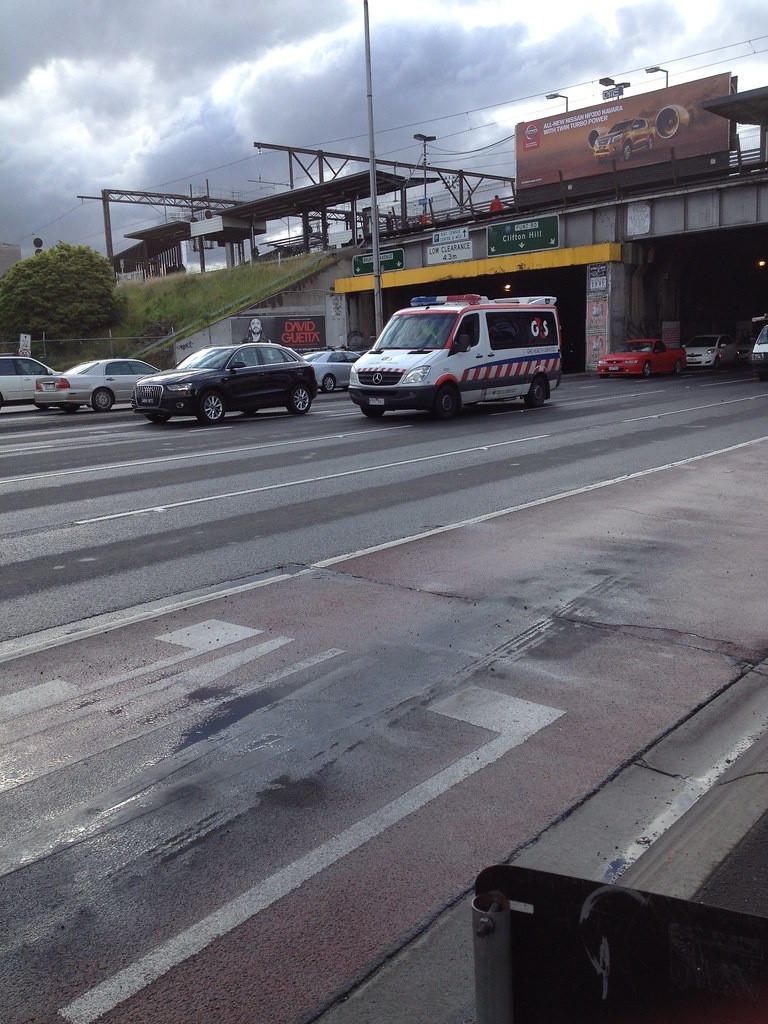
751 325 768 381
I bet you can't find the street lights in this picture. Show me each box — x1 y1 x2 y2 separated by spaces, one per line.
414 134 436 216
645 67 668 88
599 77 630 99
546 92 568 112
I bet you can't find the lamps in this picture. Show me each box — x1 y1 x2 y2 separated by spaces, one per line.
599 78 614 86
546 93 558 99
645 66 660 73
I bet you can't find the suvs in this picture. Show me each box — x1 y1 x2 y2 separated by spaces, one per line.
131 343 318 427
594 119 655 165
0 357 63 409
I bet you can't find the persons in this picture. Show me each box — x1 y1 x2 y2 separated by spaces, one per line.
242 319 272 343
490 195 502 211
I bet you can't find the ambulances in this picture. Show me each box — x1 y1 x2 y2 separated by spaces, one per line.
348 293 561 418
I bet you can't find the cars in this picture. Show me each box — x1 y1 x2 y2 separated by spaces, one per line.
682 334 758 369
302 351 361 392
34 359 163 413
597 338 687 378
735 337 767 367
